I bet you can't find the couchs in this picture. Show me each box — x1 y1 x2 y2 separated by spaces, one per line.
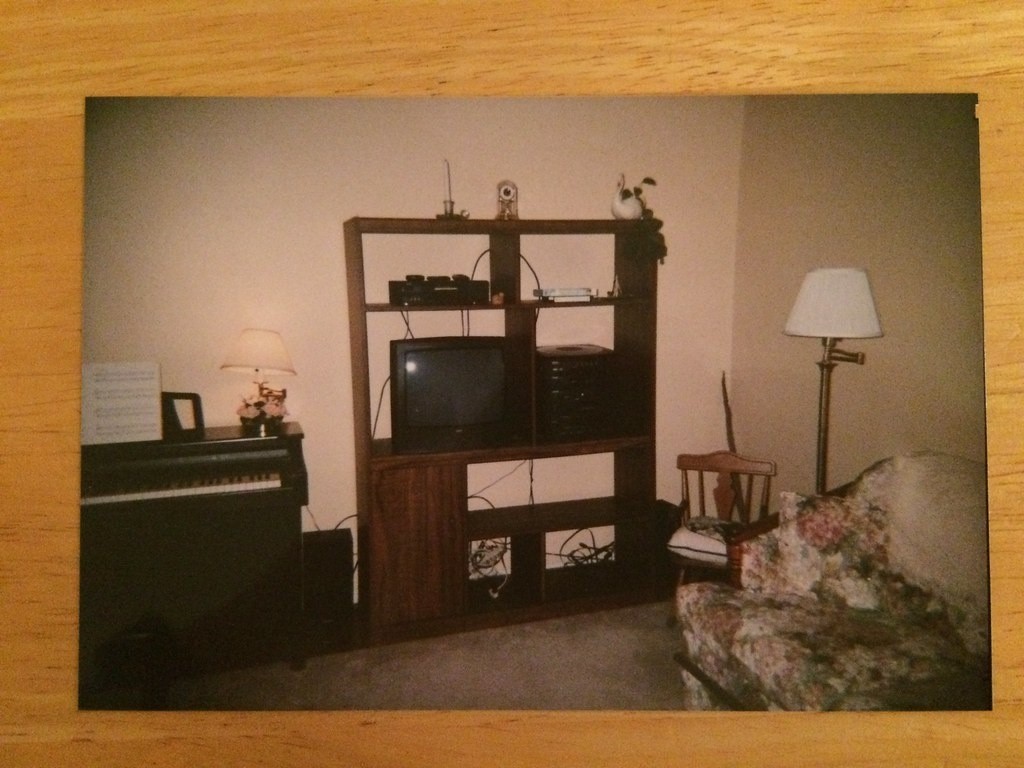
671 447 993 711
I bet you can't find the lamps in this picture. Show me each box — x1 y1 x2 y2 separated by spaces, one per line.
784 266 885 496
218 328 299 433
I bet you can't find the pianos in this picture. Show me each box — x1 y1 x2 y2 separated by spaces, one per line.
80 421 311 692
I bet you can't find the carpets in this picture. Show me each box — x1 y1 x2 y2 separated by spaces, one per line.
165 600 743 710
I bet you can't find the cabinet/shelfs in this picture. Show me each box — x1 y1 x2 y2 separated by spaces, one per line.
342 215 660 645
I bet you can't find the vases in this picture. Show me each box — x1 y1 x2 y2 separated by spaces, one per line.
240 415 262 431
261 416 283 431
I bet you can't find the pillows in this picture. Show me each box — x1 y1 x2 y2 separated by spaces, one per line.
774 489 893 612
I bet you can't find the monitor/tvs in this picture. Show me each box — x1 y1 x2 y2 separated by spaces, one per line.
388 336 523 454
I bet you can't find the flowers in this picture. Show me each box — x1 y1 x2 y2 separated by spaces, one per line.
261 399 291 418
237 395 266 418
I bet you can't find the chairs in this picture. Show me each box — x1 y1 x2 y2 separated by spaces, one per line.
664 450 778 627
